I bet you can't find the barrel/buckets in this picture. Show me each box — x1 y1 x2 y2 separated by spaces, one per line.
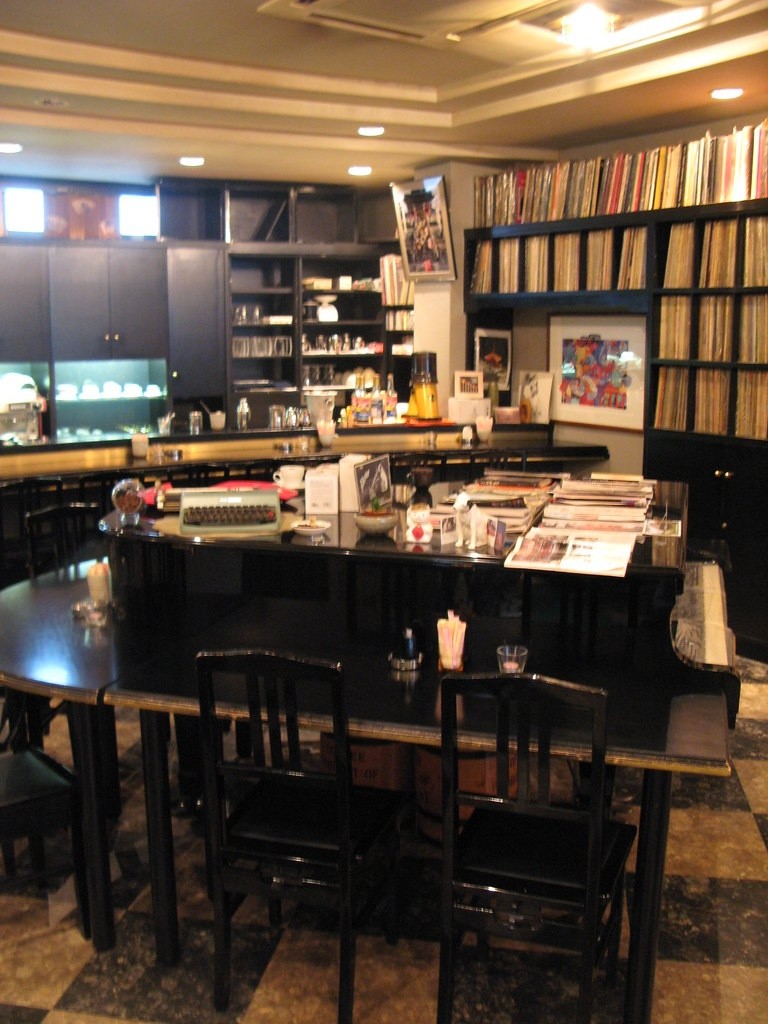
304 390 338 427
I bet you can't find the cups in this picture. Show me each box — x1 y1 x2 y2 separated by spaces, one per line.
132 434 149 457
302 364 379 386
210 413 225 430
436 624 466 669
476 416 493 443
496 645 528 674
234 304 263 324
158 418 171 434
273 465 304 485
232 333 368 357
392 485 417 503
86 574 111 606
269 404 311 429
189 411 203 434
462 425 473 443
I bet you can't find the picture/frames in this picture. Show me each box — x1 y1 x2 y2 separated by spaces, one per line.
390 175 458 283
544 313 648 434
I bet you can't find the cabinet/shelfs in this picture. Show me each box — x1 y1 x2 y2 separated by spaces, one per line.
462 198 768 663
0 176 415 395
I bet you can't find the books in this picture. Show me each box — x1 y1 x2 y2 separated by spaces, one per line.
475 119 768 229
381 254 417 330
654 293 768 440
429 465 657 577
470 215 768 287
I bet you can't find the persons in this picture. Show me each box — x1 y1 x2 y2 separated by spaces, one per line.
406 213 440 263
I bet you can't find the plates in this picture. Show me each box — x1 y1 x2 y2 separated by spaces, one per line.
273 482 306 489
291 520 332 536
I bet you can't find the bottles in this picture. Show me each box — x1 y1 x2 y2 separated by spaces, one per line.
371 375 383 423
112 480 144 527
384 373 397 421
341 405 354 429
351 373 361 405
488 381 499 416
356 376 369 422
520 400 531 423
237 398 251 430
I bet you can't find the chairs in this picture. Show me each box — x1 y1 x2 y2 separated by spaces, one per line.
1 450 637 1024
